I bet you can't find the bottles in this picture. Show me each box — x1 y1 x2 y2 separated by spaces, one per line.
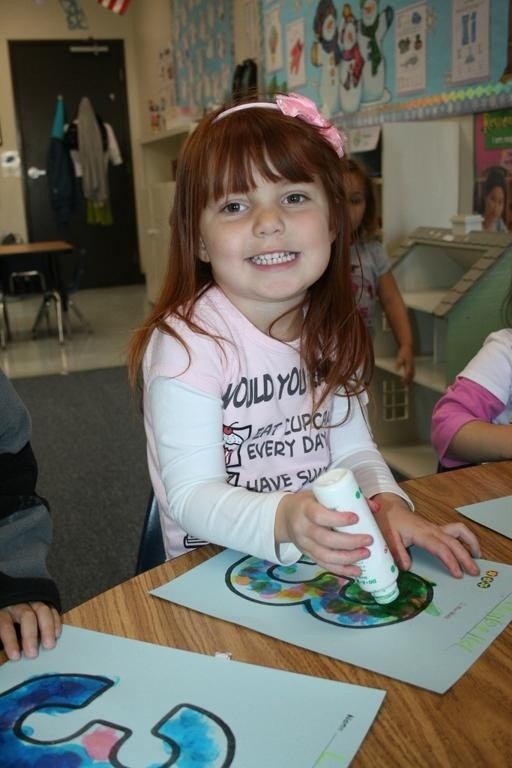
312 468 400 605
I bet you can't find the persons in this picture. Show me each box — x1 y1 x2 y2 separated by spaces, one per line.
129 91 481 580
480 171 509 234
332 161 416 385
0 370 61 663
430 286 512 470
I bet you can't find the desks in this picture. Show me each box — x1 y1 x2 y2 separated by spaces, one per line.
2 460 512 767
1 241 94 345
